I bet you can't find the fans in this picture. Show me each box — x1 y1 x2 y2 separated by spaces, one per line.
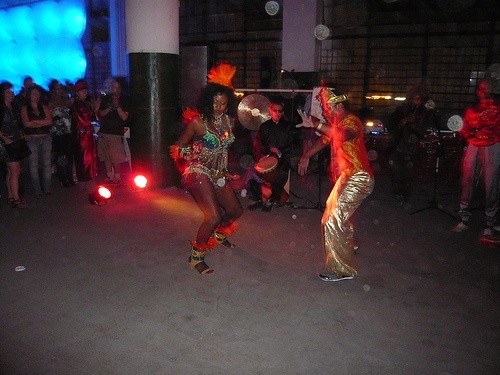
237 94 272 158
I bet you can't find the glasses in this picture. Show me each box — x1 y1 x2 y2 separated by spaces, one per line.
271 109 283 113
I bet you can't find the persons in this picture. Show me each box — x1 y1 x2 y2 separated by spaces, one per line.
0 75 130 209
386 85 435 181
452 76 500 236
292 89 376 283
248 102 294 212
171 83 244 277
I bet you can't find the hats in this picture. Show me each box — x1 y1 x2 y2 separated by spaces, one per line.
75 82 87 90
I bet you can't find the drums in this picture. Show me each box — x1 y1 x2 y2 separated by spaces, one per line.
254 156 279 181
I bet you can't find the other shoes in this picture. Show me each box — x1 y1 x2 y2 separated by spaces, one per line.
261 199 273 211
248 200 262 211
452 222 468 233
214 233 237 248
188 250 215 276
484 228 492 236
7 175 80 212
319 273 354 282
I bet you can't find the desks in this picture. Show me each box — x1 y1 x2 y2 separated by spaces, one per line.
364 134 404 196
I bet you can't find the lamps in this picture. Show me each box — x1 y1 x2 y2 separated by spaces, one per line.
88 185 111 208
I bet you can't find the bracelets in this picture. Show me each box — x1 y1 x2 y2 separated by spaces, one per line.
1 133 8 139
315 121 324 132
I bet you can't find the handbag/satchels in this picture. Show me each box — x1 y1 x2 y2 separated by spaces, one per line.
4 138 32 161
